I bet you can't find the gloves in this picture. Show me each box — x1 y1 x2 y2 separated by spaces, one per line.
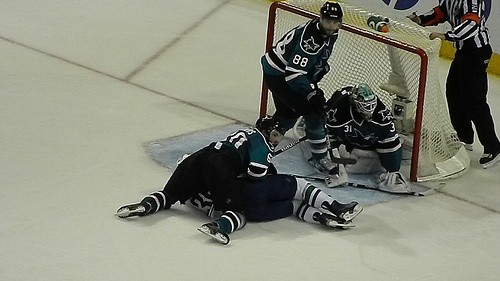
305 90 325 114
367 16 389 33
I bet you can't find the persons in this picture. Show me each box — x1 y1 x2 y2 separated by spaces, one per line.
325 82 407 187
407 0 500 169
256 2 343 178
115 114 363 245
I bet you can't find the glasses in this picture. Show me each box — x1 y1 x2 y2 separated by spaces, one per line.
322 19 342 29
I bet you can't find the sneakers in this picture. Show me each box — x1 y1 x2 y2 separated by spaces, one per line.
197 221 231 245
479 149 500 169
312 200 363 229
114 201 152 218
450 133 474 151
313 158 340 179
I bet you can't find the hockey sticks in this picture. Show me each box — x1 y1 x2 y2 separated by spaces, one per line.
274 135 309 156
314 82 358 165
287 174 446 197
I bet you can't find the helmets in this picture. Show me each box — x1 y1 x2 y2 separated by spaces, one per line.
255 115 284 149
351 82 377 119
320 2 343 20
